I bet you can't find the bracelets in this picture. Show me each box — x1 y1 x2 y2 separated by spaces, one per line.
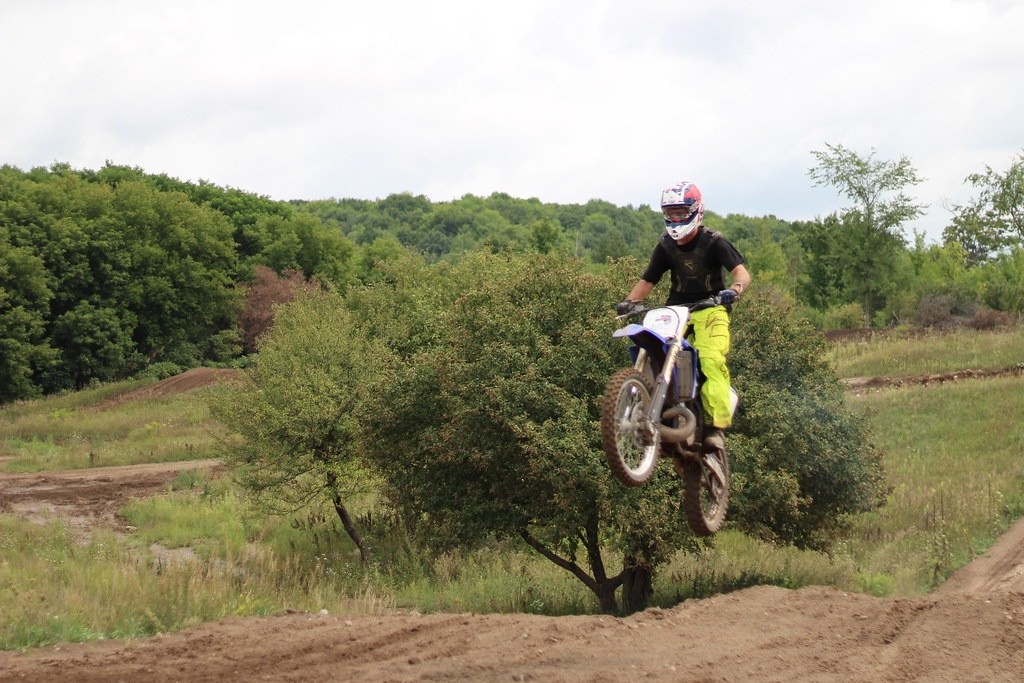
731 282 742 295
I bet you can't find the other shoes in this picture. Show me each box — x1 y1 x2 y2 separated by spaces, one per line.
702 428 725 450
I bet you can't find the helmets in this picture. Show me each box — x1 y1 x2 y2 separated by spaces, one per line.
661 181 703 241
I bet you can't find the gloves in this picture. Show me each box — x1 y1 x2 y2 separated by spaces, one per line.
717 289 739 305
616 299 635 315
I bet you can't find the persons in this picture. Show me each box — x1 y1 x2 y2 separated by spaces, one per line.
616 182 751 451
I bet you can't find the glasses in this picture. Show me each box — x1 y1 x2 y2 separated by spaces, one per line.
661 198 701 223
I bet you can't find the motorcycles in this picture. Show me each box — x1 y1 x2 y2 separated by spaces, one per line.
601 292 740 538
